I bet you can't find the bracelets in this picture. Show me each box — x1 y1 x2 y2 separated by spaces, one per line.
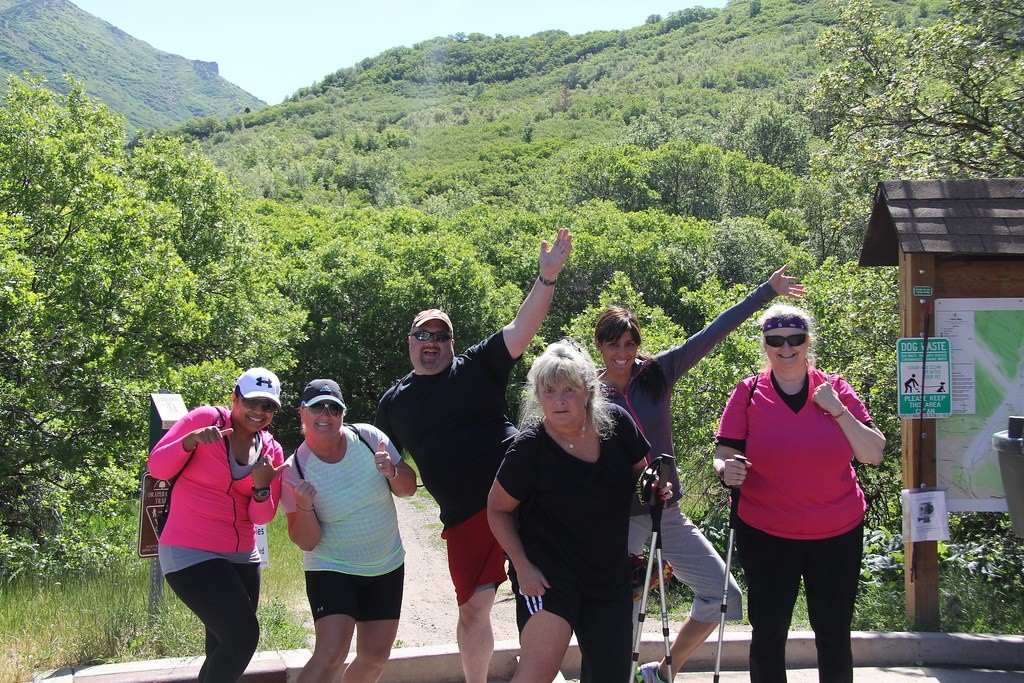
539 273 557 285
834 405 848 418
718 467 725 482
296 504 312 511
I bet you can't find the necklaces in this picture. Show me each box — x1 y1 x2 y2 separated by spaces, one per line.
563 423 586 448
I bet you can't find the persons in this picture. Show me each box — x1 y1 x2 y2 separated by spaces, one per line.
714 303 885 683
148 365 290 683
593 265 807 683
487 336 673 683
374 227 573 683
279 379 417 683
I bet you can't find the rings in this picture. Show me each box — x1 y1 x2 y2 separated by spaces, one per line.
379 462 384 469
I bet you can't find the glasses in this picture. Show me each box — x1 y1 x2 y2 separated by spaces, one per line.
411 331 452 343
238 393 277 413
765 333 807 347
304 403 343 416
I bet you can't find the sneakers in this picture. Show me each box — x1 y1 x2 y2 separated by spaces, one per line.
636 661 674 683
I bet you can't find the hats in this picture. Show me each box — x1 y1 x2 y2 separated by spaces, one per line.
234 367 282 408
410 308 453 338
301 379 346 410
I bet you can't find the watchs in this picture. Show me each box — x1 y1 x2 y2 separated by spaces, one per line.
385 465 399 480
252 485 270 497
